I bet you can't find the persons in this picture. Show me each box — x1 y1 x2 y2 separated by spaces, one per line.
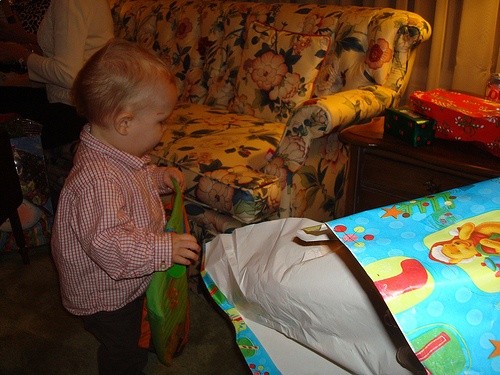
51 38 202 375
0 0 115 214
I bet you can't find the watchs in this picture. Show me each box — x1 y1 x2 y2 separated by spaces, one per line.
19 48 30 69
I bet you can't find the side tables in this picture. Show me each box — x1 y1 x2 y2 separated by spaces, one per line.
336 104 500 218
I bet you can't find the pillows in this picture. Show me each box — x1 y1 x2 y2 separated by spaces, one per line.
229 20 332 124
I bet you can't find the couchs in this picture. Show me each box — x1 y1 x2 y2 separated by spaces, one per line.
35 0 432 256
